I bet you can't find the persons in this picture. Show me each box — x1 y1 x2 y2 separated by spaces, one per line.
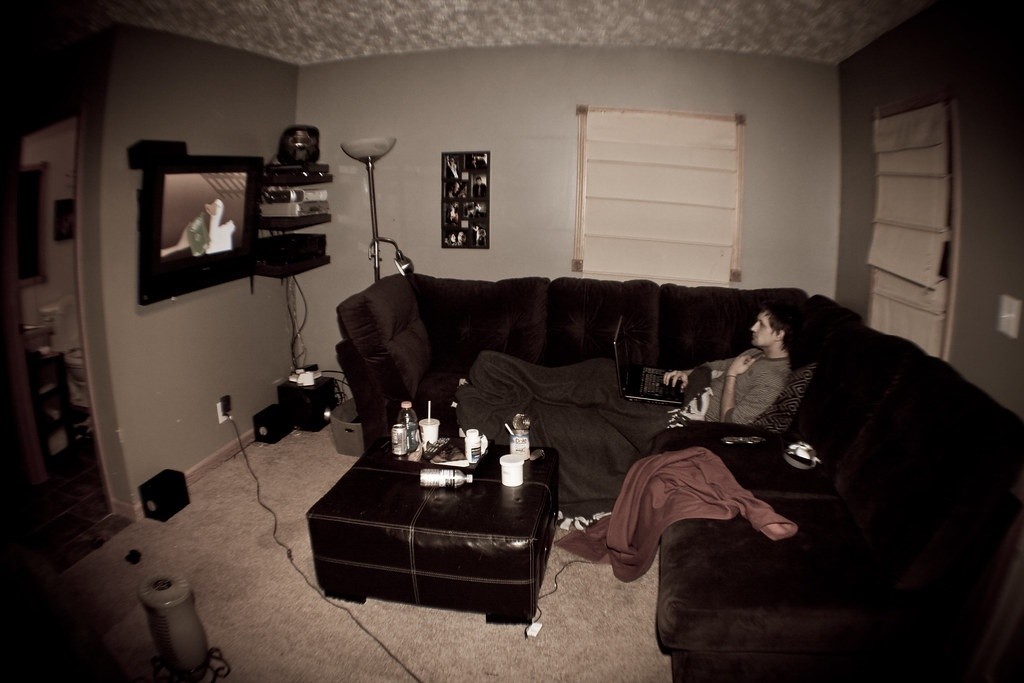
663 302 803 425
442 153 487 248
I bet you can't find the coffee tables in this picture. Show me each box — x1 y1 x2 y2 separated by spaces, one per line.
313 447 561 628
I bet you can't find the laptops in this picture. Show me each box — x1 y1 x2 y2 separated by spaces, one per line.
612 315 685 407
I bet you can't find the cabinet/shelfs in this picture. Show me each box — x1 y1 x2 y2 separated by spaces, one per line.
34 350 70 464
254 172 334 279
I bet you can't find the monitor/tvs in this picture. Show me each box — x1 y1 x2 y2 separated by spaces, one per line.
136 154 264 307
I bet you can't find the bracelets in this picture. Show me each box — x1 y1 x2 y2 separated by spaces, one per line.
724 375 737 379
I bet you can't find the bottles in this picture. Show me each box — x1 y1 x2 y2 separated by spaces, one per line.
419 469 473 487
397 401 419 452
465 429 482 463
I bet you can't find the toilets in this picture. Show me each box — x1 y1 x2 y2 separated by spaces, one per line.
65 351 89 408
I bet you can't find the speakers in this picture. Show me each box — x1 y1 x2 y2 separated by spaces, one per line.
138 469 190 522
252 376 336 443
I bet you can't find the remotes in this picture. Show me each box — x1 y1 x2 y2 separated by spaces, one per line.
721 436 766 446
424 437 452 459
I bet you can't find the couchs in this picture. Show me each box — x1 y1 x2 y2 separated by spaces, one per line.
334 275 1024 683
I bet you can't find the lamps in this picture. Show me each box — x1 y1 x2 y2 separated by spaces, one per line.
341 136 414 280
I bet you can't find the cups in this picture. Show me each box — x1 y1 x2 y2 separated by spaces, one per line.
500 454 523 487
419 419 440 452
510 430 530 460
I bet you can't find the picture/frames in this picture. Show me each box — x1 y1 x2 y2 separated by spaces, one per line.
441 151 491 250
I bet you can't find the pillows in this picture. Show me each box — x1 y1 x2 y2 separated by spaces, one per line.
725 359 817 445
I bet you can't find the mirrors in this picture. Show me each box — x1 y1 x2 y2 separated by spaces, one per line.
16 160 50 290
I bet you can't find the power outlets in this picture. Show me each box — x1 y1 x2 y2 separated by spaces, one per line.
216 401 230 425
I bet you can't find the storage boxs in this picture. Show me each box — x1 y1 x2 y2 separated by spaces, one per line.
332 400 363 457
